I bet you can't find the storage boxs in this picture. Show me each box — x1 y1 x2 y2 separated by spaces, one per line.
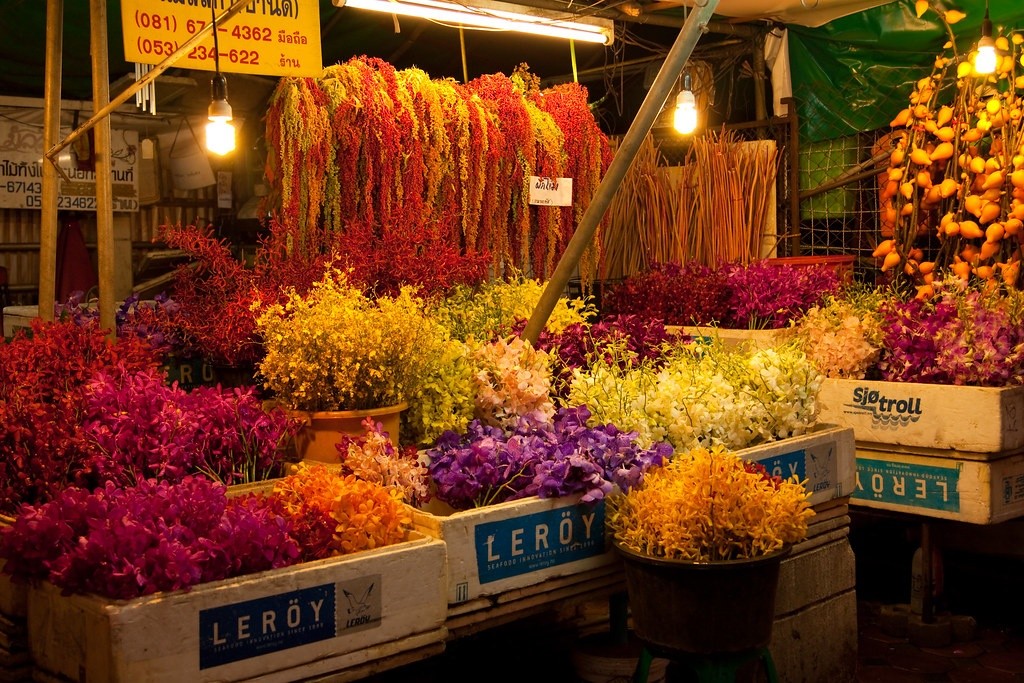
815 375 1023 454
663 325 787 355
30 485 448 683
849 449 1024 524
408 454 615 603
734 427 857 507
0 476 291 616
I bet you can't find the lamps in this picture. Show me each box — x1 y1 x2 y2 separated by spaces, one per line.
332 0 616 46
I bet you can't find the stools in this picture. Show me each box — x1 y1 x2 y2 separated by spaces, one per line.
628 648 779 683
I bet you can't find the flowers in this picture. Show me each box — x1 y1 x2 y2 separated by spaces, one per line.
0 56 1024 597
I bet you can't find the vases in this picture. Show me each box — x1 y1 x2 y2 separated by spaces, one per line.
283 401 408 466
770 256 855 286
613 543 792 652
